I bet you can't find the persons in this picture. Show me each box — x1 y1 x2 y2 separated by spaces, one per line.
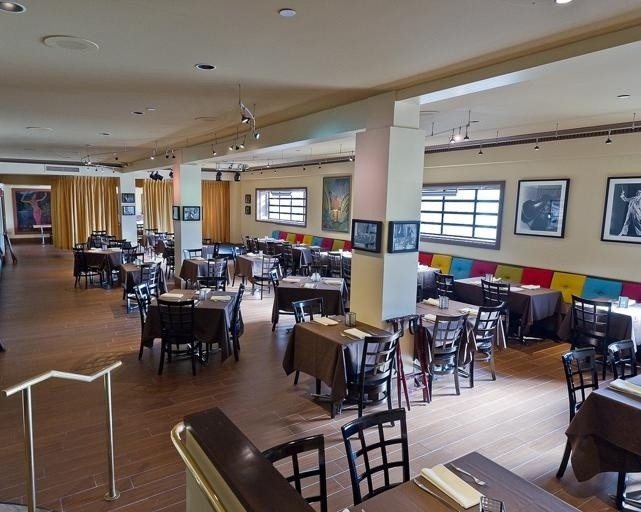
19 190 47 226
619 187 640 238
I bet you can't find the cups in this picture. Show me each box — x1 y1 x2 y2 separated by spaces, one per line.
619 296 629 308
198 290 207 302
259 250 264 257
344 312 356 327
102 244 108 250
486 273 494 283
265 235 269 241
438 295 451 310
135 258 142 266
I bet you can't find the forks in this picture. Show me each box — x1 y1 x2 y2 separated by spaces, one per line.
449 461 490 487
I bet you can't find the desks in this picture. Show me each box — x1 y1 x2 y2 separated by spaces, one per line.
33 223 52 245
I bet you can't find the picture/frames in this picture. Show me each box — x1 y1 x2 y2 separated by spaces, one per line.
514 178 571 239
322 174 421 254
244 194 252 216
601 176 641 245
121 193 136 215
171 205 201 222
12 188 51 235
